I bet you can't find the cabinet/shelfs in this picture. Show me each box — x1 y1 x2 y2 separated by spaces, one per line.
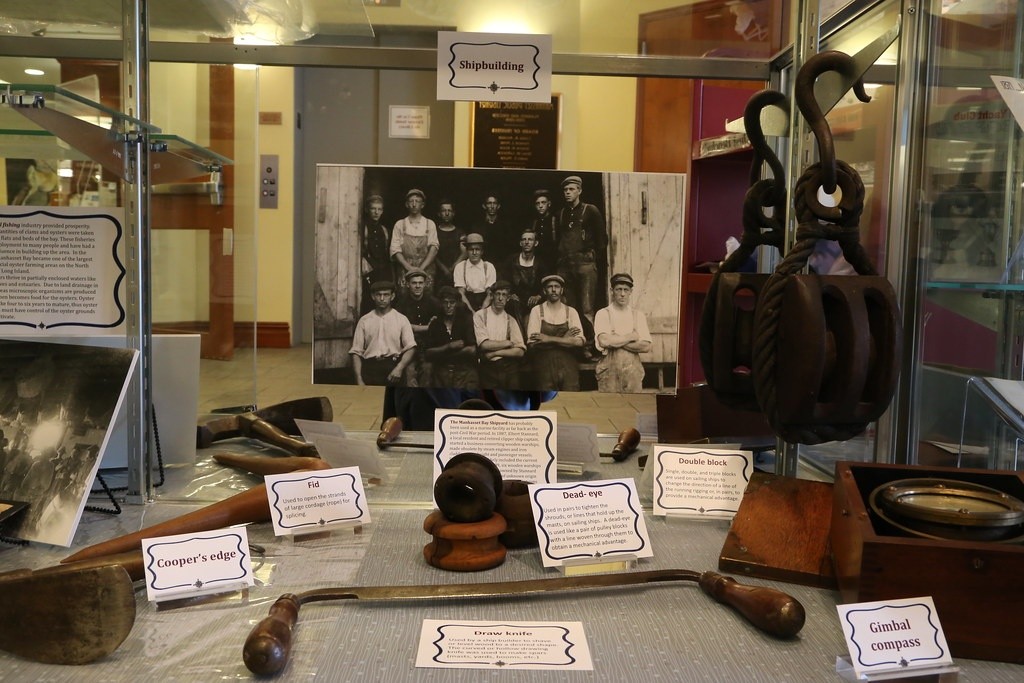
631 0 784 390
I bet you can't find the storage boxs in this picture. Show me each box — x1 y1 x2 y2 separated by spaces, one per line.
829 461 1024 666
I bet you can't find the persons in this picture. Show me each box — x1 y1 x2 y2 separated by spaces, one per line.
0 428 100 541
348 175 605 432
593 274 652 394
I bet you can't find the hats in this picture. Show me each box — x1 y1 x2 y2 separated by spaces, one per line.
541 274 565 286
491 280 513 291
560 176 582 187
405 189 425 198
462 233 486 246
609 273 634 288
369 280 395 291
438 285 462 300
404 269 427 279
